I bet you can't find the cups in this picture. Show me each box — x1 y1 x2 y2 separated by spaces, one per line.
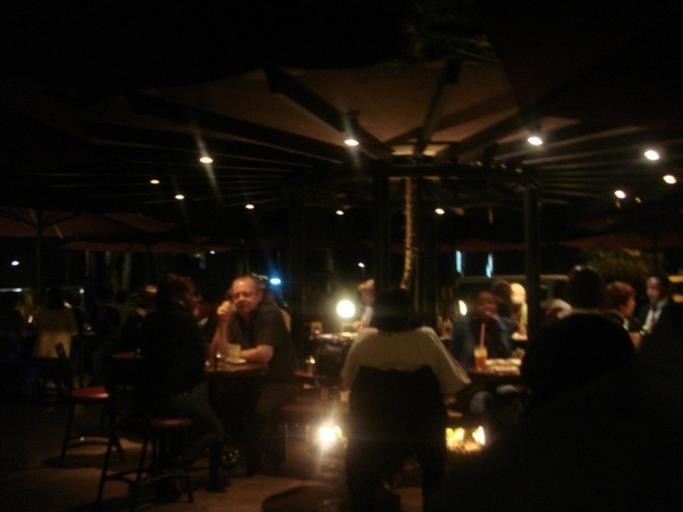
473 346 487 369
225 344 241 365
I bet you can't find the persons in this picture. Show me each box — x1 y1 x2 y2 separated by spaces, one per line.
1 265 682 510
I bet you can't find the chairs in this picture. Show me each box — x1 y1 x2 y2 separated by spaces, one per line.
52 289 683 512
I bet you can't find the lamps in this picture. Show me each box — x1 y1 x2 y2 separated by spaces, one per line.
197 136 214 164
644 146 660 162
527 124 544 150
342 110 363 148
663 169 679 185
